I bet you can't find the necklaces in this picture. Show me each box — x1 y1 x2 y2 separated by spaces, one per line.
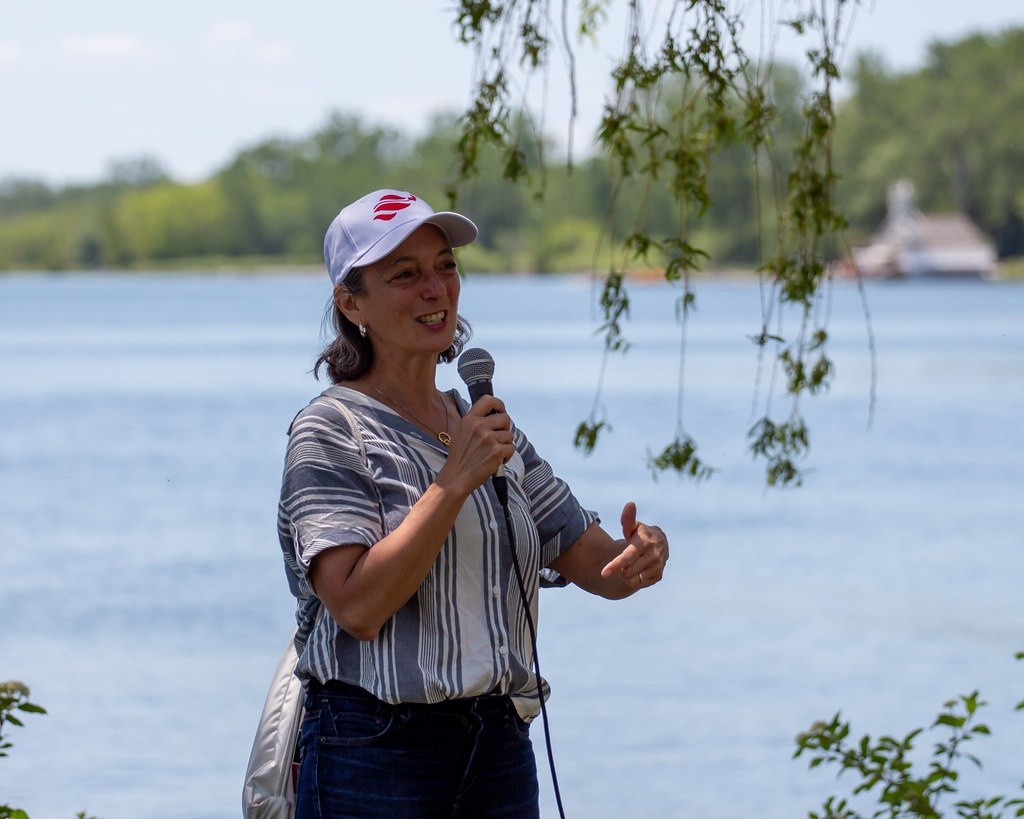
358 374 453 447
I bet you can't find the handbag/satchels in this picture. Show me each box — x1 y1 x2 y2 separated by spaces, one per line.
241 397 366 818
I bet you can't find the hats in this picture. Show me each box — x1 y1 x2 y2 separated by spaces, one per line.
323 189 479 285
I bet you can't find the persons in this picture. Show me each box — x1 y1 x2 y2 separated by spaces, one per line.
275 186 670 819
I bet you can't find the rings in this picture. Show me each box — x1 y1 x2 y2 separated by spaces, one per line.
638 572 644 586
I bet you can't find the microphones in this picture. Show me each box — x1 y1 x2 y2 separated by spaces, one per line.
457 347 509 504
